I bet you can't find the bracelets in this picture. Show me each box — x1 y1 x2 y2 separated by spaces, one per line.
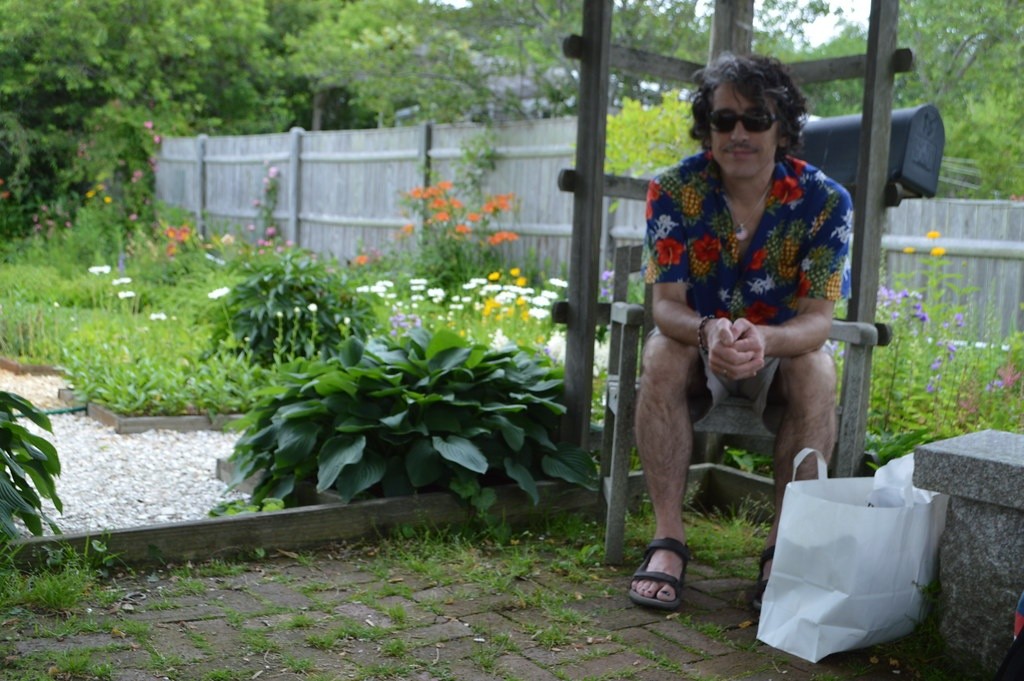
697 311 716 357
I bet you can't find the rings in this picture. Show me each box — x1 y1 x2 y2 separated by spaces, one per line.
723 366 730 379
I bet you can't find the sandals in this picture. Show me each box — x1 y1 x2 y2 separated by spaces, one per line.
627 538 692 611
752 545 782 610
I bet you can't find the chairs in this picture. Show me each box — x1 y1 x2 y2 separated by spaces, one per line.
597 243 878 567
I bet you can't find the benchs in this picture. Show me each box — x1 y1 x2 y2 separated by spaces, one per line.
912 429 1024 681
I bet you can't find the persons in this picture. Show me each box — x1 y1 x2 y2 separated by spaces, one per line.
628 56 858 621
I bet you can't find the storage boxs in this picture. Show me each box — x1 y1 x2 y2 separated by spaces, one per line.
790 104 945 198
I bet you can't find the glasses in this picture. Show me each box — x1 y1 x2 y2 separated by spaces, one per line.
708 104 780 132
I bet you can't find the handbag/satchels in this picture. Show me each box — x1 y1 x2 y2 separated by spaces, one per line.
756 447 948 664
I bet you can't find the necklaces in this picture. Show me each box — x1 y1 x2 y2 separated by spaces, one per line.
723 179 775 241
